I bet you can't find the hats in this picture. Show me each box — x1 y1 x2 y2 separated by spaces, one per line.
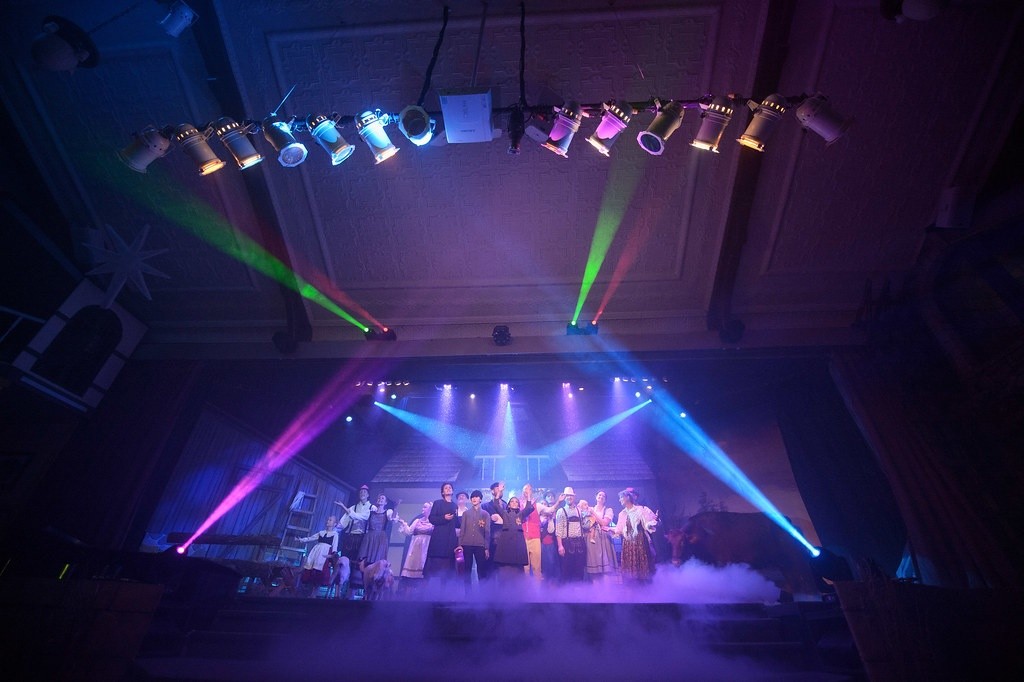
563 487 575 495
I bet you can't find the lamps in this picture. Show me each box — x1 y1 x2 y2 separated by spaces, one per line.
305 112 355 166
566 321 598 335
115 126 170 174
152 546 188 588
155 0 199 38
174 123 226 176
689 95 735 155
212 116 265 172
397 105 437 147
796 92 855 147
735 95 787 152
807 545 854 602
492 326 511 347
637 97 685 156
540 100 581 159
363 328 397 342
260 113 308 167
355 109 400 164
584 101 636 159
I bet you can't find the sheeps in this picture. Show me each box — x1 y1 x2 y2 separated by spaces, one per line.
354 556 392 601
322 551 350 599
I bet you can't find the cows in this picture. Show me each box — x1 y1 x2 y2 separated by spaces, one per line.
663 511 799 597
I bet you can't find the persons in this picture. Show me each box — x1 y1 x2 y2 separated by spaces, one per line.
399 481 660 599
332 484 397 567
297 515 339 597
334 494 401 597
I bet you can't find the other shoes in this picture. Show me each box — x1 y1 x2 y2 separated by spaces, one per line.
590 539 595 544
